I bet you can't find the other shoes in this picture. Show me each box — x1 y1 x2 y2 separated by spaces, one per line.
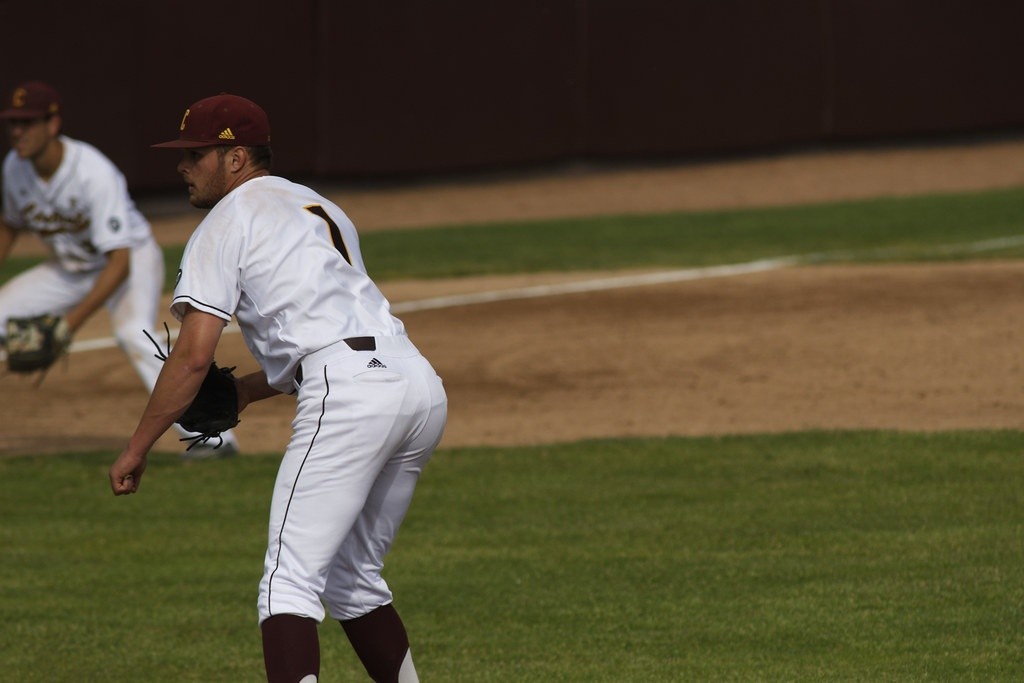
186 444 237 461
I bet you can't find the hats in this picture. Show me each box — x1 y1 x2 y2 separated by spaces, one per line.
150 92 271 149
0 81 60 118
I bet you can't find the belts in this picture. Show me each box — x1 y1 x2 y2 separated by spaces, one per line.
295 336 376 385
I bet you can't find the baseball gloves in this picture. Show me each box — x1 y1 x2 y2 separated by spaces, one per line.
0 312 72 377
175 359 239 438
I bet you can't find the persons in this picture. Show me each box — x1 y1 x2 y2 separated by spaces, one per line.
110 92 448 683
0 80 239 461
